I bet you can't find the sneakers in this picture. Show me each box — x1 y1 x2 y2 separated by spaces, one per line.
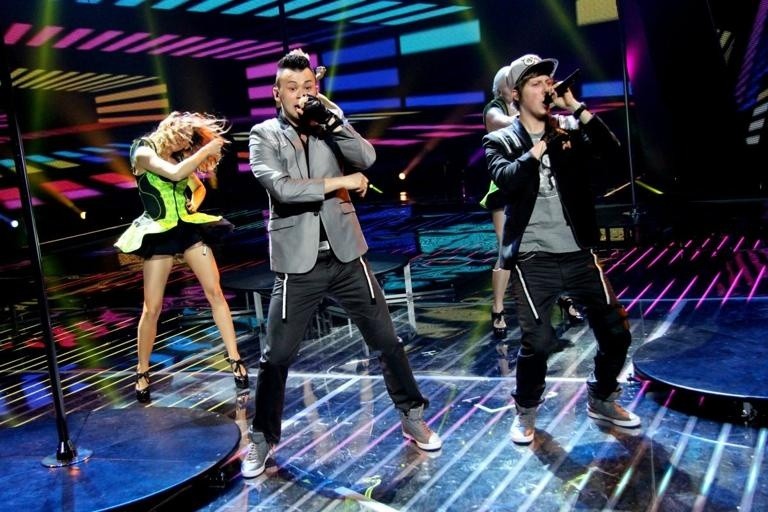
510 403 540 445
586 387 641 428
241 426 277 479
401 404 442 451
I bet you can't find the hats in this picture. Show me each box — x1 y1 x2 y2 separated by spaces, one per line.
505 54 558 92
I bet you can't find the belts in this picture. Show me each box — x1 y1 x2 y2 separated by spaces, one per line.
318 241 331 251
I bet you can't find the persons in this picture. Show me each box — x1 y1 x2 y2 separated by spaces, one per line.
482 64 585 338
240 48 441 480
481 53 641 444
114 110 248 392
301 362 376 511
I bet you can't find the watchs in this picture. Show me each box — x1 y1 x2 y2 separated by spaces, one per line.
573 102 587 120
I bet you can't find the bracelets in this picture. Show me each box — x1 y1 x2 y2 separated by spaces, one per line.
326 114 343 133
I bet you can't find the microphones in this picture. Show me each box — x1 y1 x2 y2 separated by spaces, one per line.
544 66 585 106
303 99 320 112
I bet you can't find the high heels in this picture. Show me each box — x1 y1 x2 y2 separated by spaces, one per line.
134 371 151 403
558 297 584 323
229 358 249 388
491 310 508 339
